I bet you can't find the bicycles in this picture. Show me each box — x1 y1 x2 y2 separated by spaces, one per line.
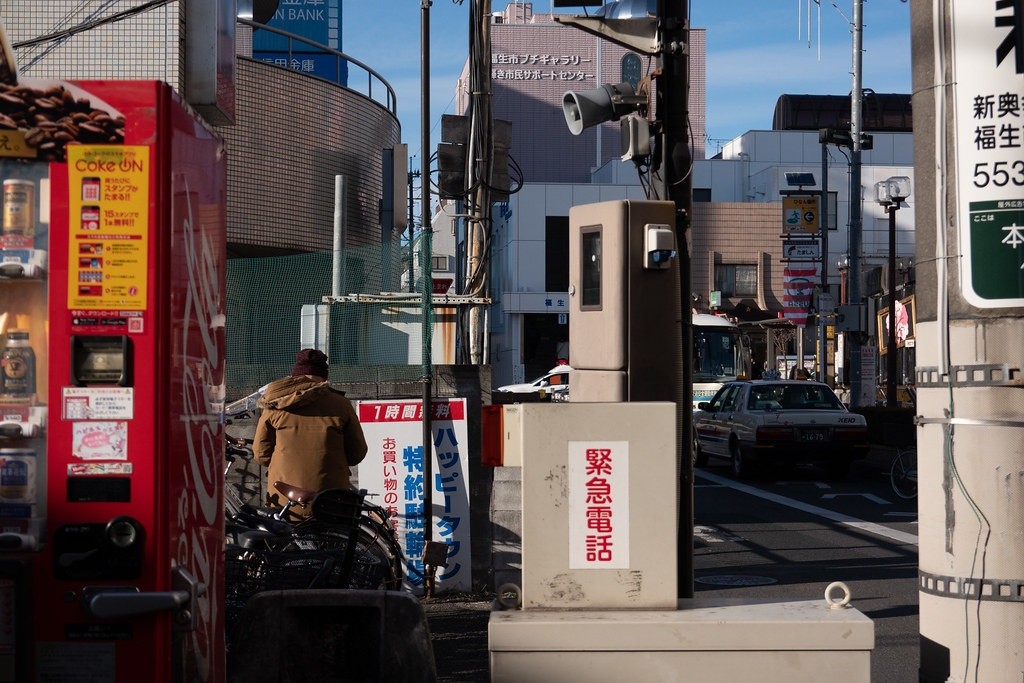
881 431 917 500
225 430 406 647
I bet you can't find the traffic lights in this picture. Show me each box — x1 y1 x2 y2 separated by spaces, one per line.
618 115 651 162
553 13 659 57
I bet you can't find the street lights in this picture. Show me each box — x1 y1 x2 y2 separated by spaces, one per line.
874 176 913 448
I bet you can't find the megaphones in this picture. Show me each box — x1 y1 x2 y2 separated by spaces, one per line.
562 80 638 136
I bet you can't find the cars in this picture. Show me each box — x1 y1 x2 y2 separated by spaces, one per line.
494 364 569 405
692 382 783 412
691 377 871 480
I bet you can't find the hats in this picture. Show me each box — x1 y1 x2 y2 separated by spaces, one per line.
292 348 329 379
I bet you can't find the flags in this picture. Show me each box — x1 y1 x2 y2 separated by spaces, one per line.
781 267 818 328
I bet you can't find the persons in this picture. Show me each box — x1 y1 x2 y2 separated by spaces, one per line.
250 348 367 522
